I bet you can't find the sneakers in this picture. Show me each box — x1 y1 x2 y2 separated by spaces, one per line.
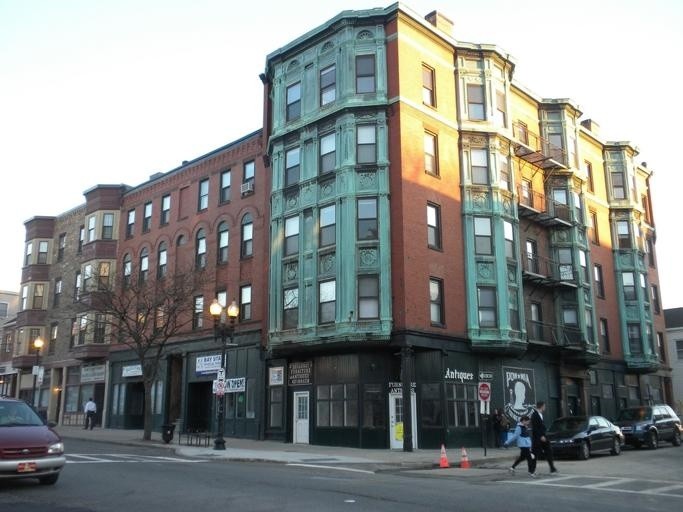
552 469 560 475
507 466 537 478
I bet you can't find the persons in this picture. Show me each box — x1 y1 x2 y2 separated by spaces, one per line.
531 401 561 477
493 408 508 449
82 398 97 429
504 416 533 475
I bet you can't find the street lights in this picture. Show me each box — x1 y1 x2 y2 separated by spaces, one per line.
208 294 241 452
30 334 45 411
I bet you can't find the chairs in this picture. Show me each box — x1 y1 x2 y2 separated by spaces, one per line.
178 424 212 446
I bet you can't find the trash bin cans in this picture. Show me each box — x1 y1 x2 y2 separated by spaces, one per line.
162 424 177 443
498 427 509 448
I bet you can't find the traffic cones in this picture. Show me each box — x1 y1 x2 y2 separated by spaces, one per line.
439 443 451 468
459 446 470 469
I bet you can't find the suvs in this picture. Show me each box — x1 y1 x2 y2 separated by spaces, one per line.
610 404 682 450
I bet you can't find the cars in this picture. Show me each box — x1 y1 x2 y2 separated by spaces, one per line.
538 415 626 461
1 394 68 487
